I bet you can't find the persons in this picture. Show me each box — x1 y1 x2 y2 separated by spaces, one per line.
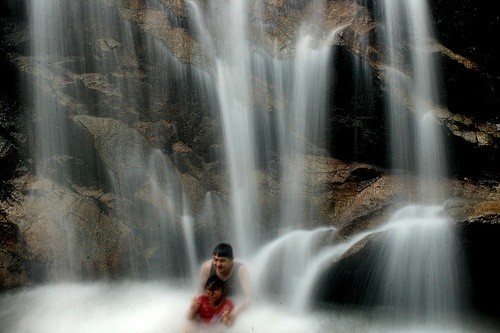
199 241 252 300
189 276 237 327
